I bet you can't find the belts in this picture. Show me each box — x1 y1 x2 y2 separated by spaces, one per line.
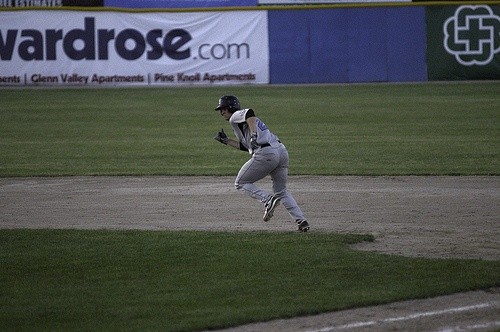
261 140 282 148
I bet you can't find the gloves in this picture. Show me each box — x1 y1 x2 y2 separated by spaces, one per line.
214 128 228 145
248 132 259 155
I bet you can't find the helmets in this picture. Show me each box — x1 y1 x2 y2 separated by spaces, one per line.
214 95 241 110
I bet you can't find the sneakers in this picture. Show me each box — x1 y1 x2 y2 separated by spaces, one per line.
296 221 310 233
263 196 280 222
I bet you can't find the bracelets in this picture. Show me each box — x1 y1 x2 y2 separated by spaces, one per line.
250 133 258 140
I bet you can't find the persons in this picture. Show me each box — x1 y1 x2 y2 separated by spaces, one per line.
214 96 310 232
